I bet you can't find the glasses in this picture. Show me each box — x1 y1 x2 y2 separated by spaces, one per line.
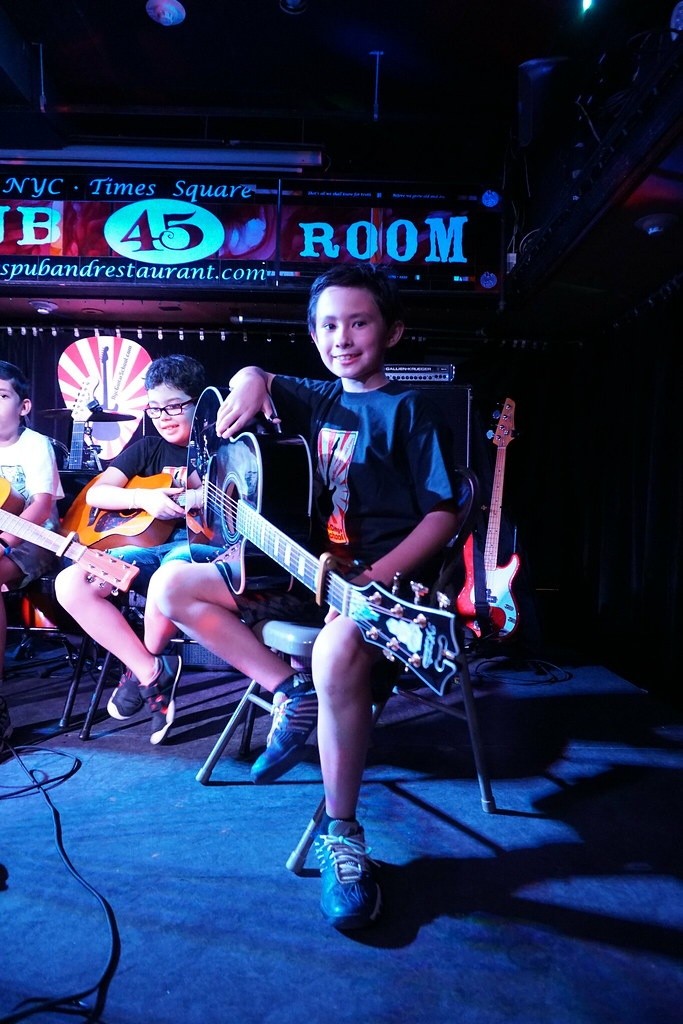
145 397 197 419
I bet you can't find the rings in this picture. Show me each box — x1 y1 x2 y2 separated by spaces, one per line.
267 411 276 424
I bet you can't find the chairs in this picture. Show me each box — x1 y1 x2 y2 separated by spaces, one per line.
191 465 497 871
58 566 290 760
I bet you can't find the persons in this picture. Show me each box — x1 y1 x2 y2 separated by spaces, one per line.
55 357 215 745
151 266 461 930
0 361 65 753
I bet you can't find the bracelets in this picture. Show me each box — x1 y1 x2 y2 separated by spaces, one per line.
129 487 140 509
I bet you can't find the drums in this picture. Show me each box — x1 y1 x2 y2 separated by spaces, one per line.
41 433 68 471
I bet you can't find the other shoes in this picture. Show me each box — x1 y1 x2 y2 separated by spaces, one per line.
0 701 12 752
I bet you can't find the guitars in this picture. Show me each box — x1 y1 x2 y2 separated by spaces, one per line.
68 377 100 469
185 386 463 699
457 396 521 643
0 474 141 596
62 471 187 552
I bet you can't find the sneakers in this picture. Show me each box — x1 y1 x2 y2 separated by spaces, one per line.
138 655 182 745
315 821 382 926
250 689 318 784
106 666 145 720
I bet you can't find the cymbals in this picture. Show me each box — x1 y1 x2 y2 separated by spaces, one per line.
38 407 138 422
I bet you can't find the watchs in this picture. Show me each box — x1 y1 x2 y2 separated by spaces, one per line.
0 538 11 557
